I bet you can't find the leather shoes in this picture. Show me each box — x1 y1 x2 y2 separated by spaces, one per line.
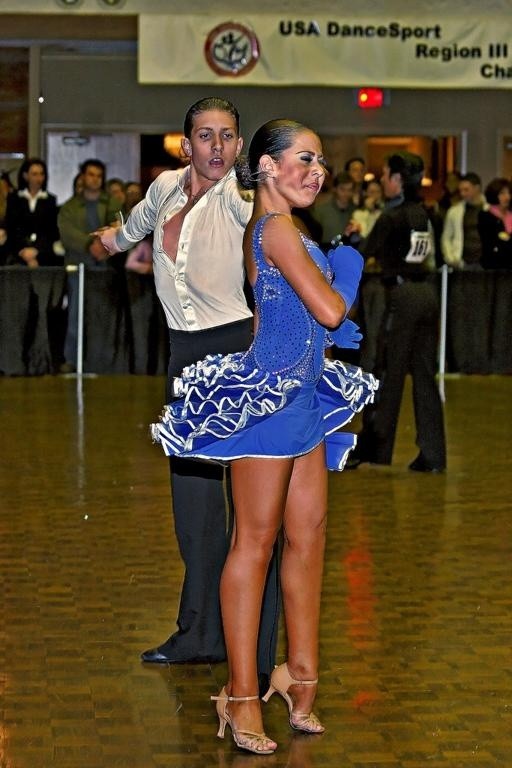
142 648 182 664
411 450 434 471
343 452 375 470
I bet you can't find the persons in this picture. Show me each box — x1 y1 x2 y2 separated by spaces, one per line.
314 154 512 381
88 96 284 699
344 151 448 473
0 156 171 382
196 115 366 756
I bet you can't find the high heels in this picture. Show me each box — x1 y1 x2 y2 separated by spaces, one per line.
209 685 277 756
260 661 326 735
